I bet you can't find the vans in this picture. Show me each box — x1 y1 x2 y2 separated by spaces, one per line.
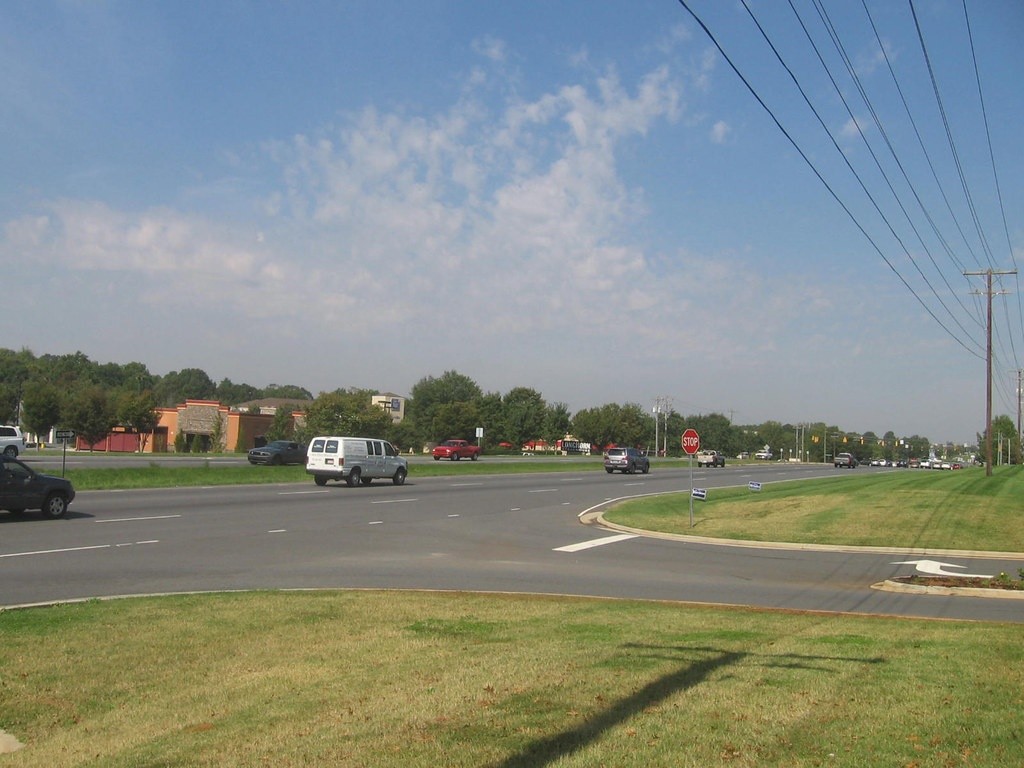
304 436 409 488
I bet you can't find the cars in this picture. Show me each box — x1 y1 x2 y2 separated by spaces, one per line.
247 441 307 465
737 452 749 459
755 450 773 460
0 453 75 520
861 457 962 471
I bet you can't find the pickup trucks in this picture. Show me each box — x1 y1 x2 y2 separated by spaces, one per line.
834 452 859 468
432 439 481 461
698 449 725 468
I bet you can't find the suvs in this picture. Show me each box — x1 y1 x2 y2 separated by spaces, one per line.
603 448 650 473
0 425 26 459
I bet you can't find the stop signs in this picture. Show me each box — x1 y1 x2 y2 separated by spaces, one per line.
681 429 700 455
